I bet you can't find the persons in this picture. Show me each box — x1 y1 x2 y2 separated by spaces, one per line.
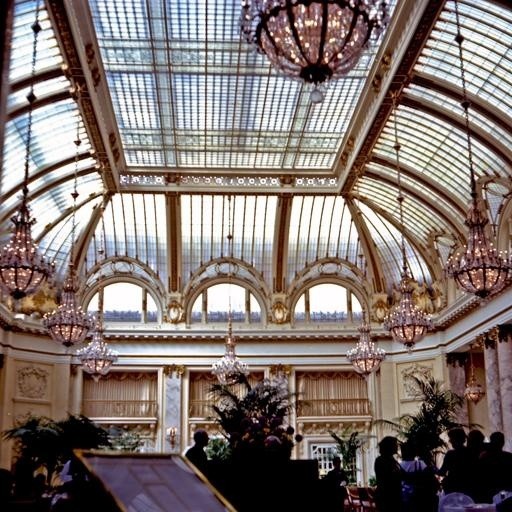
373 427 512 511
185 430 211 473
328 455 349 512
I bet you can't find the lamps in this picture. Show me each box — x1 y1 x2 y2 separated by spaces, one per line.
460 342 490 408
234 0 401 107
385 38 431 346
164 425 181 451
2 111 120 388
445 0 511 303
346 166 385 379
209 192 248 390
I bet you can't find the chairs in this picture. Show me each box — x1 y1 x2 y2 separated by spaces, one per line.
438 491 512 512
344 483 379 512
